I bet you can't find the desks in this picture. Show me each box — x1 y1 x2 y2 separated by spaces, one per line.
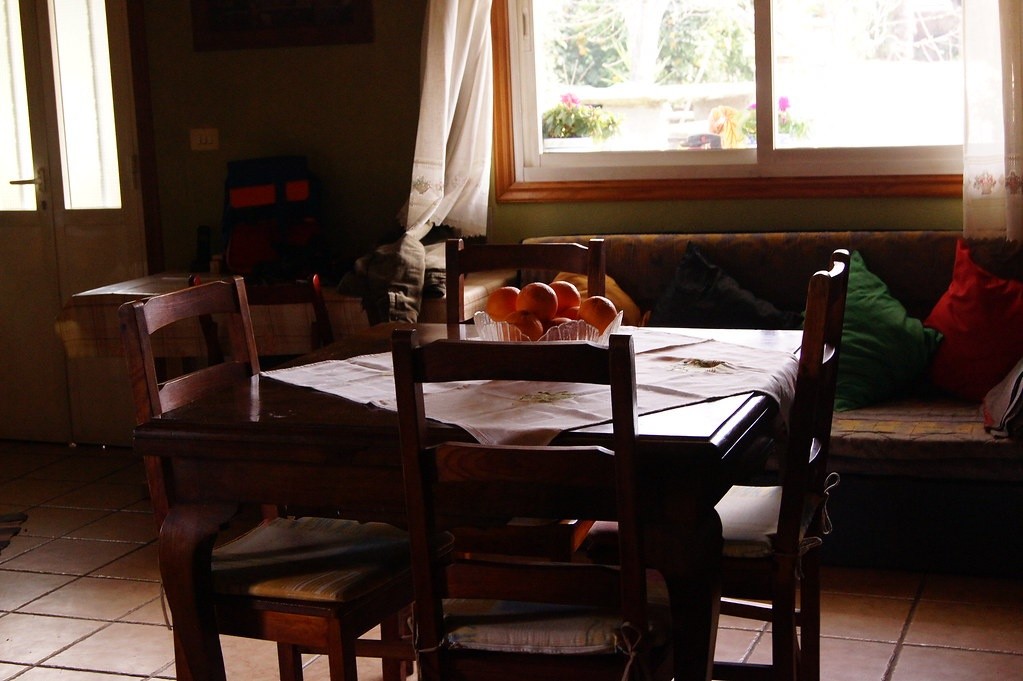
61 269 404 458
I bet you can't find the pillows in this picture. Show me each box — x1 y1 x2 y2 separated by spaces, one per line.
549 268 643 325
920 237 1023 405
801 248 946 413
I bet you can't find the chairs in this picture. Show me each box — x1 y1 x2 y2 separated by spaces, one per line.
713 244 852 680
443 238 607 338
113 271 412 681
390 324 722 681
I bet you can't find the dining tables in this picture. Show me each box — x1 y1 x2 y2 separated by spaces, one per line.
260 316 813 675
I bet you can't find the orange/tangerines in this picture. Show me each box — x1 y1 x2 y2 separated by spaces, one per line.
485 281 617 343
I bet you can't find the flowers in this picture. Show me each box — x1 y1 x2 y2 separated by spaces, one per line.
540 93 619 140
744 95 806 137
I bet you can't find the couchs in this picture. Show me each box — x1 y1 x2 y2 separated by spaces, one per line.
432 227 1023 478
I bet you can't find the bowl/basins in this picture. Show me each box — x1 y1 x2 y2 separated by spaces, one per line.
473 310 625 346
